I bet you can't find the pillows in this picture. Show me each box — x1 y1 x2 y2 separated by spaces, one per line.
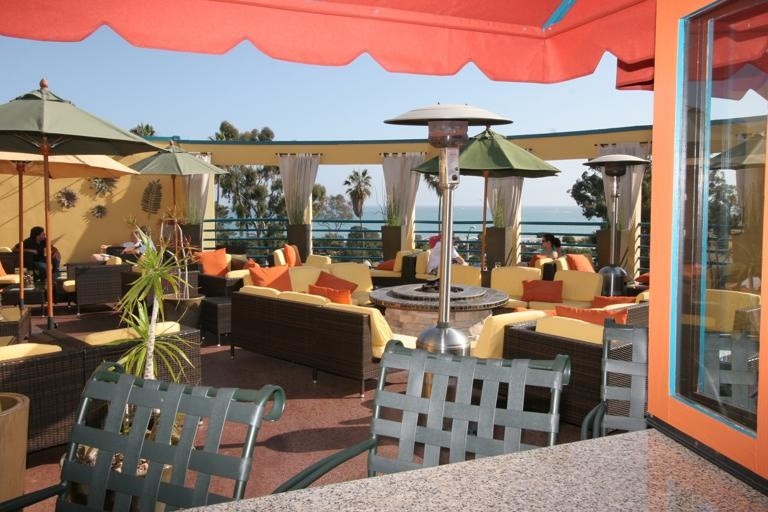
375 258 396 270
285 243 297 267
588 295 637 309
520 278 563 303
566 253 596 274
248 262 289 287
314 270 358 295
555 305 628 326
192 247 230 279
308 283 351 304
266 268 293 292
634 271 649 285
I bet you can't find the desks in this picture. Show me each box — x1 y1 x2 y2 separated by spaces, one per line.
368 279 507 338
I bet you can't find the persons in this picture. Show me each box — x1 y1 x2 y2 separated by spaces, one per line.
740 269 761 290
10 225 62 284
534 231 564 261
121 225 159 256
100 229 142 250
728 194 744 231
424 233 467 277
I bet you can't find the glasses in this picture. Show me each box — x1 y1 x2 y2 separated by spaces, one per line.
541 240 549 243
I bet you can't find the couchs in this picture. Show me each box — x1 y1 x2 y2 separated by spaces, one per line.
201 297 232 347
436 262 604 316
0 246 61 281
42 314 203 433
230 285 419 398
193 264 240 297
62 256 135 316
263 246 287 266
705 289 762 337
232 263 387 317
1 333 82 462
502 317 648 434
369 249 439 288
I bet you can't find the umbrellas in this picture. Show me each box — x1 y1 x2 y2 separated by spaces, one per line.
407 127 563 272
0 148 143 317
127 141 234 322
0 77 176 330
709 130 766 170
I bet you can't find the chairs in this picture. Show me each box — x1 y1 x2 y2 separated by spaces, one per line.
2 361 285 512
580 319 648 441
270 339 572 496
703 328 759 417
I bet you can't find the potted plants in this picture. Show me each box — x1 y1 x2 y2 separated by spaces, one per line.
59 218 204 512
124 203 205 334
